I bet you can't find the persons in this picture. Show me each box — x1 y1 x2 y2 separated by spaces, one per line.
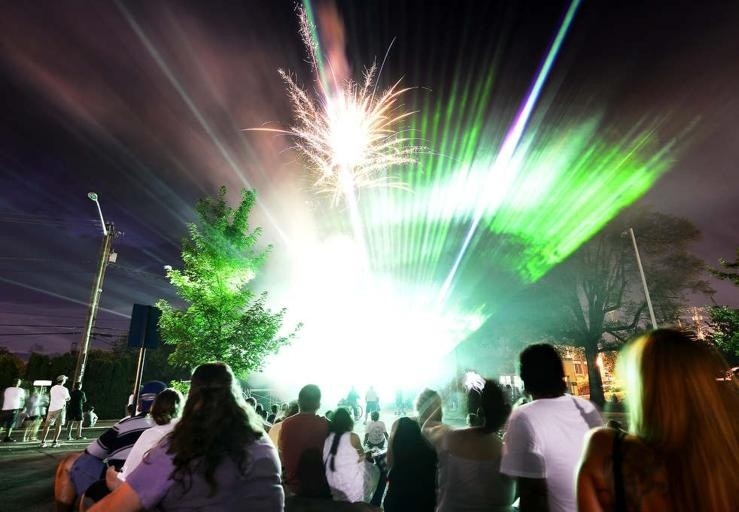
573 325 739 510
1 372 100 448
501 343 606 512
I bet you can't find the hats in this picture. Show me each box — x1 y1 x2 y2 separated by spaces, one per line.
126 393 135 408
138 380 168 402
57 374 68 383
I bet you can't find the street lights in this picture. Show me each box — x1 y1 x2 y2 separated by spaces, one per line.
69 185 124 401
618 224 659 331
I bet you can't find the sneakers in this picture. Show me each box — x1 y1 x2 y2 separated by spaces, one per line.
51 442 61 449
75 436 87 441
39 441 48 447
67 437 75 441
0 436 38 442
